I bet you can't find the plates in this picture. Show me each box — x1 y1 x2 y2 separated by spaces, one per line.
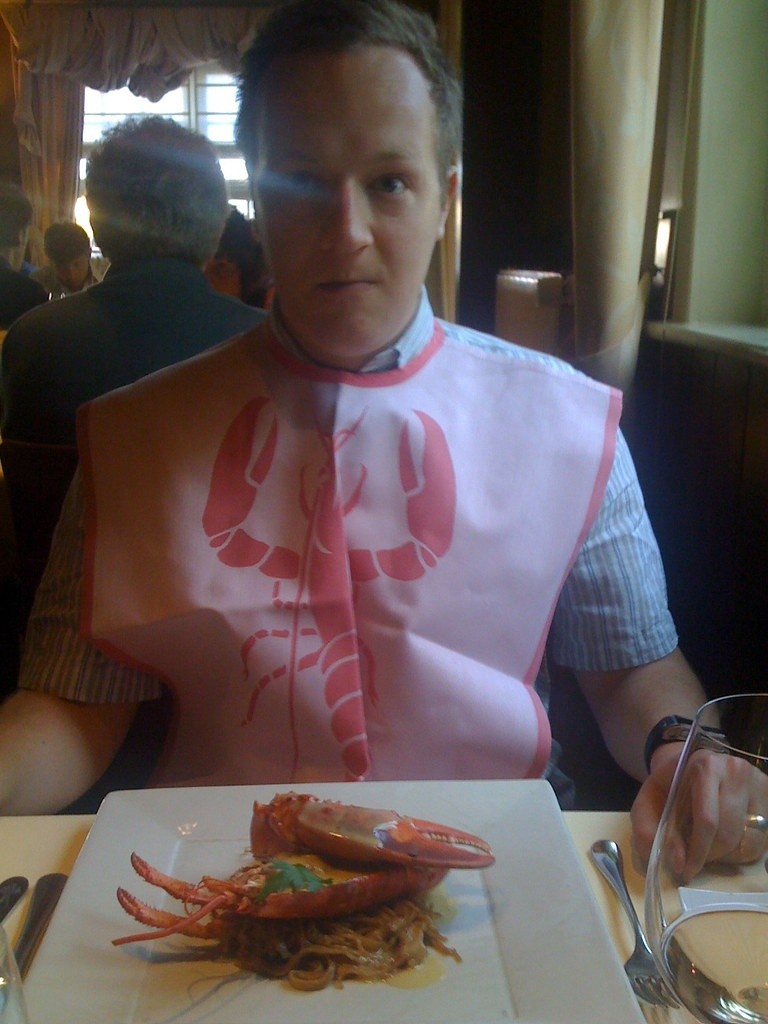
22 777 648 1024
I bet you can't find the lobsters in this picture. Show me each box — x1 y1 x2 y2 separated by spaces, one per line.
107 789 496 947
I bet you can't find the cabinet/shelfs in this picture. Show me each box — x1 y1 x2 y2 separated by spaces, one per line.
648 346 766 576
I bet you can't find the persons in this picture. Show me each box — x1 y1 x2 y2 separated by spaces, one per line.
0 121 271 702
0 0 766 882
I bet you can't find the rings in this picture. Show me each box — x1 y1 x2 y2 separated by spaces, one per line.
746 815 767 831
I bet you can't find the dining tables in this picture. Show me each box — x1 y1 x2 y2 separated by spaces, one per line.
0 810 768 1023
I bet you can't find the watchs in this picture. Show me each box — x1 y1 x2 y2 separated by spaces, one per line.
647 714 727 773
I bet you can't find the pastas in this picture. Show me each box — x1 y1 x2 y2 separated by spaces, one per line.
198 881 465 992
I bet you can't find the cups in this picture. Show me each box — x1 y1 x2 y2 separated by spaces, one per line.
48 290 71 302
0 927 33 1024
645 694 768 1024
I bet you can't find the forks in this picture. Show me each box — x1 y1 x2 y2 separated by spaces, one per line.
591 838 682 1013
